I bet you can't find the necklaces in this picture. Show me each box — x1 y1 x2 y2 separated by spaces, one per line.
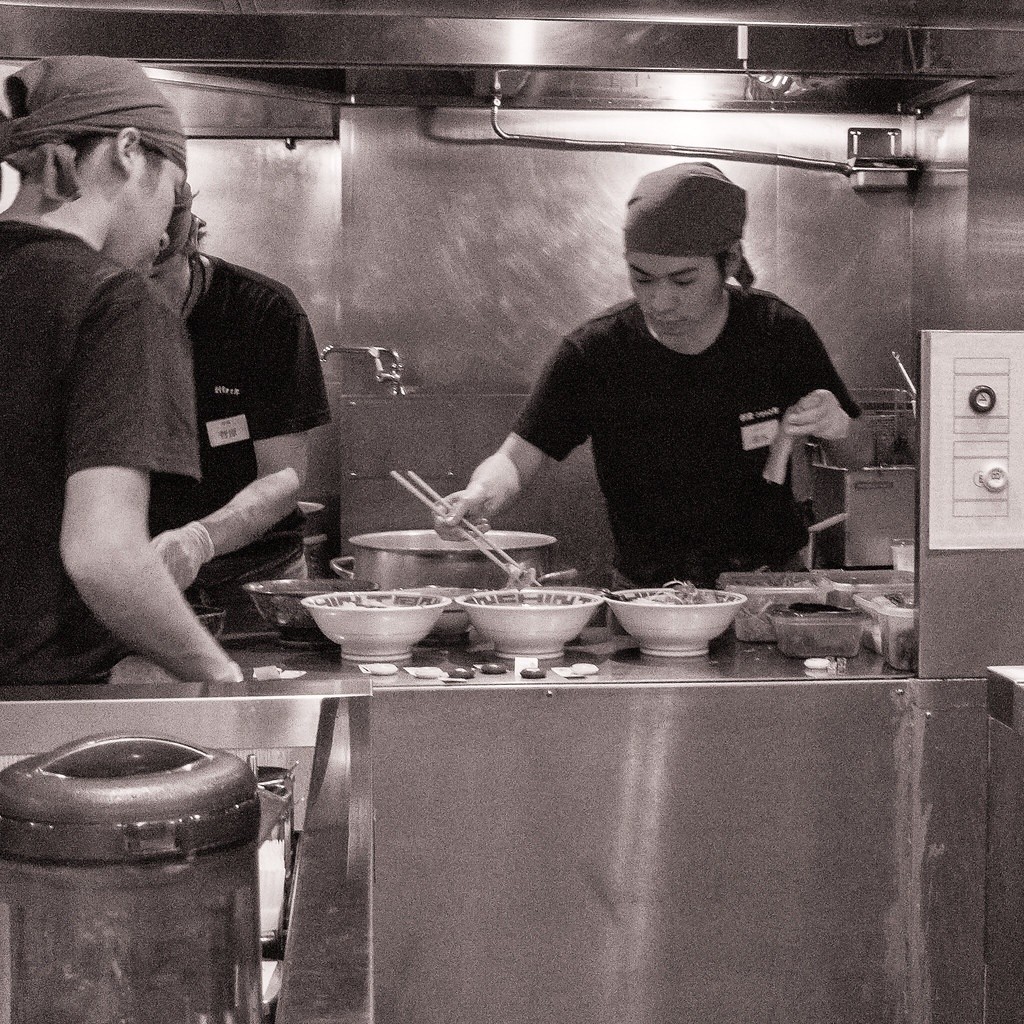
180 257 206 322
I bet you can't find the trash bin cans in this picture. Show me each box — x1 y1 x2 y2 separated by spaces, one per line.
0 732 264 1024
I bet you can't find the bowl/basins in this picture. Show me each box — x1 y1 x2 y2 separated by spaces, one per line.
190 603 226 640
455 587 608 659
240 578 381 649
300 588 488 663
603 589 747 658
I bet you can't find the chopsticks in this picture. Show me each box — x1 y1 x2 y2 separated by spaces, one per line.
389 470 546 589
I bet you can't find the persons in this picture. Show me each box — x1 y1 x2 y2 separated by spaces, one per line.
0 55 244 684
434 161 862 634
146 183 333 630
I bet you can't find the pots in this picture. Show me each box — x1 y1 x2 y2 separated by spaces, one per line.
329 528 579 588
